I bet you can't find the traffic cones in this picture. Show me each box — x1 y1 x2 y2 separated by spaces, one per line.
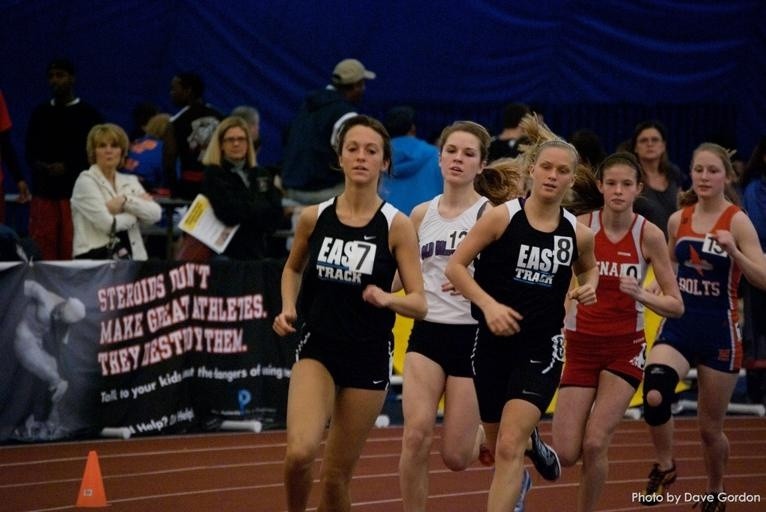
73 448 113 508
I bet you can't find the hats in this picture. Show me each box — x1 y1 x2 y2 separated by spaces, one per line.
334 59 376 83
46 58 76 77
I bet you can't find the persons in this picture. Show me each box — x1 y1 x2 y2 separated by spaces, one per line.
267 111 430 511
551 150 689 512
23 59 109 264
273 58 373 187
0 92 32 228
638 142 766 511
443 108 601 511
66 98 765 292
390 120 498 511
158 73 221 198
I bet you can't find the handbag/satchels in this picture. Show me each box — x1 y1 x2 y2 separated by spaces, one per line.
105 236 133 260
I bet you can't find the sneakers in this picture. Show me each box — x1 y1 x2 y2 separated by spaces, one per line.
475 424 496 467
513 468 533 512
696 489 729 512
639 460 678 505
522 424 562 482
